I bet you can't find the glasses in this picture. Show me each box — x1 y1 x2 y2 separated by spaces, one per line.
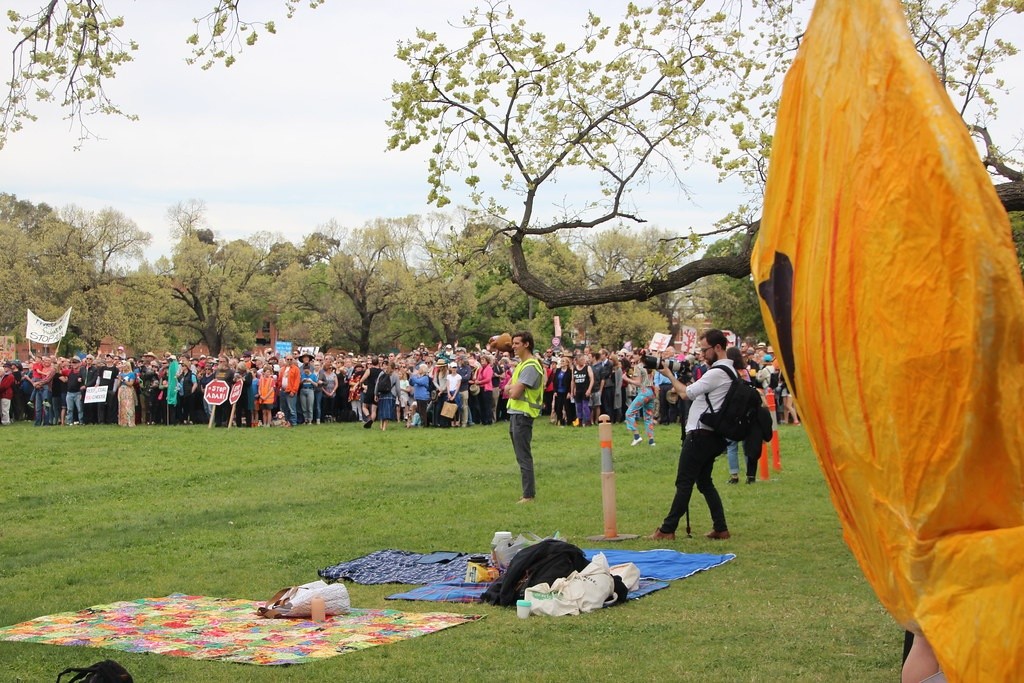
58 356 92 365
286 353 419 364
700 346 714 354
617 354 624 357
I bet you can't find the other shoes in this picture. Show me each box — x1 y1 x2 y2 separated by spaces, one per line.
649 441 655 447
744 477 757 485
704 529 730 540
631 437 643 447
726 478 739 484
641 526 676 541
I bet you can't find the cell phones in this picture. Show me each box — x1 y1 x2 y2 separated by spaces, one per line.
409 366 413 372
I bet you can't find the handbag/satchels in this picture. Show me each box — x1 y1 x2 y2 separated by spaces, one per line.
255 578 352 618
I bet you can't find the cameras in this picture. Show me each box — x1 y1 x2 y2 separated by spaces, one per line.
642 354 690 378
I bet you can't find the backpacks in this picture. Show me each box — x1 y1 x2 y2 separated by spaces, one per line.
377 373 397 395
699 365 763 442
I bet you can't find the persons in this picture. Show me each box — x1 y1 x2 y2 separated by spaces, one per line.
0 339 805 430
503 332 544 505
641 329 775 541
622 347 657 449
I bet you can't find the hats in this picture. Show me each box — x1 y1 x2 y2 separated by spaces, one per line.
767 346 775 353
763 355 772 363
758 342 767 348
7 346 283 371
666 390 679 405
469 384 480 396
450 362 457 368
434 358 447 368
695 347 703 354
297 351 314 363
419 343 487 362
